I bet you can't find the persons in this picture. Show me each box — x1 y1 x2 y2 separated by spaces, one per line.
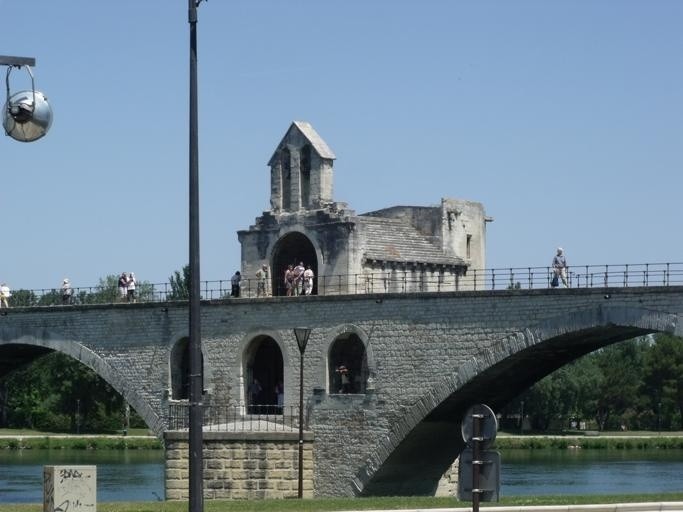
127 273 138 303
334 365 353 394
254 264 270 298
246 377 262 414
294 261 306 296
118 271 133 302
61 276 74 304
550 247 570 287
284 265 295 296
0 281 12 309
231 270 243 299
302 265 316 296
274 379 284 414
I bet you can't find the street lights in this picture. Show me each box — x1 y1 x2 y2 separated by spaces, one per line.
292 326 312 497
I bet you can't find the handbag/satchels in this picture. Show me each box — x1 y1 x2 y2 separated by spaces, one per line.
550 276 559 286
